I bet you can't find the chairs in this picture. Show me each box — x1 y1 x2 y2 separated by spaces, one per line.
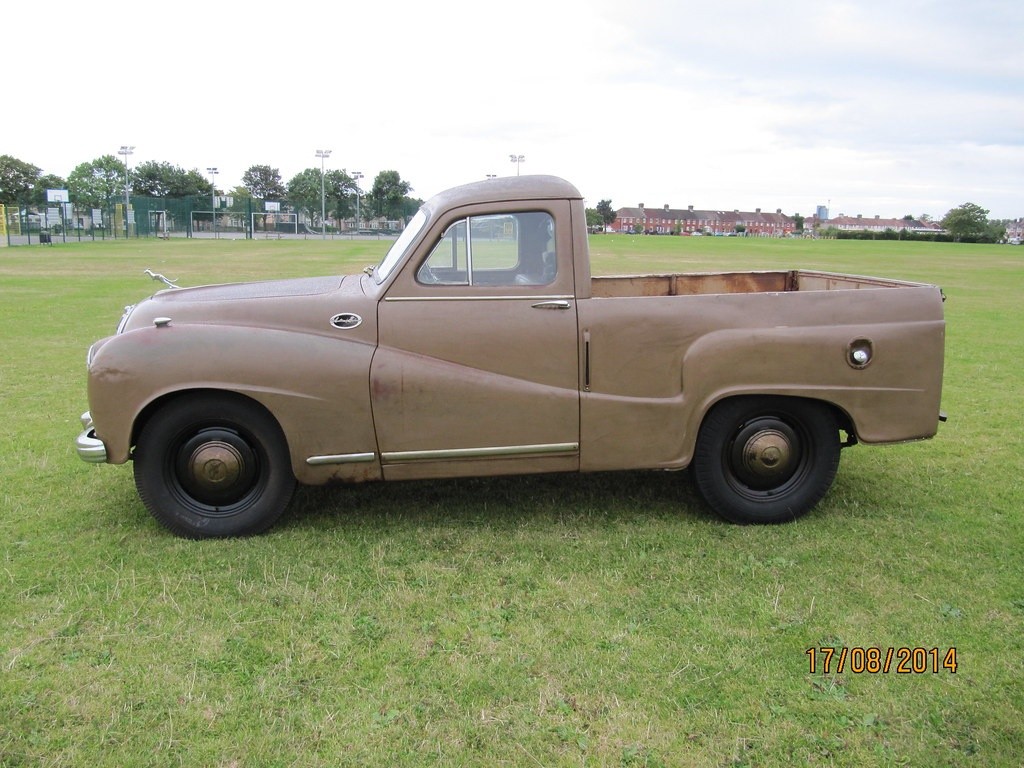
515 253 556 285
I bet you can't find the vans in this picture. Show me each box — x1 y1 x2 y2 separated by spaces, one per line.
602 227 616 234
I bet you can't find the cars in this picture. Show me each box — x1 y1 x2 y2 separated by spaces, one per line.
691 232 703 235
626 229 640 234
725 232 737 236
358 226 392 236
1012 237 1021 244
641 230 669 235
714 232 723 236
679 231 689 236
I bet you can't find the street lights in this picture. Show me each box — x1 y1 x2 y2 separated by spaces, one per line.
314 149 332 239
510 154 525 175
486 175 497 242
206 167 219 241
352 171 365 235
118 145 136 237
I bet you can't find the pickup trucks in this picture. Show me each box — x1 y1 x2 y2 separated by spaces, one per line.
76 173 947 540
